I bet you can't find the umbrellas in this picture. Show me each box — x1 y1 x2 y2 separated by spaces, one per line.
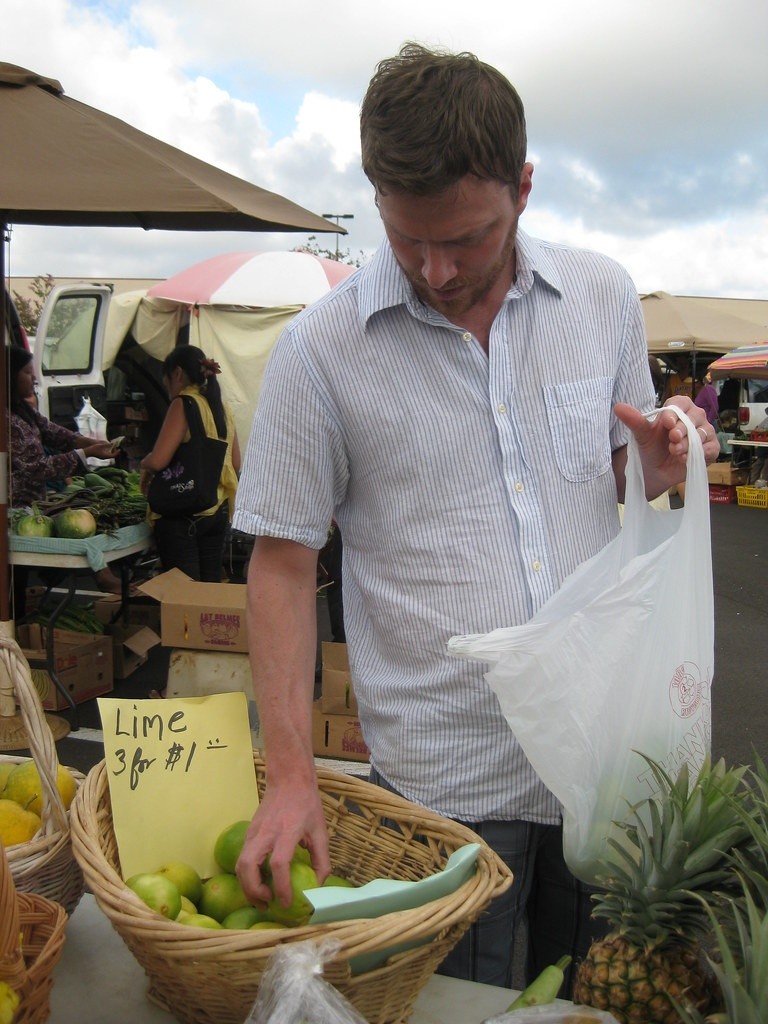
0 63 349 305
707 340 768 378
146 250 359 304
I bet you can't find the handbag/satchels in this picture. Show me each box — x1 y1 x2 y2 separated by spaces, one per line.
715 431 736 455
445 405 715 893
147 395 229 517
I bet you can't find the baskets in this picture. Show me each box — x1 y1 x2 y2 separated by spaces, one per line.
69 749 514 1024
0 636 87 916
0 850 68 1024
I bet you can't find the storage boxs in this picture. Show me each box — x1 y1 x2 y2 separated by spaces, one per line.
709 485 736 504
706 463 748 485
312 697 371 764
16 624 114 711
96 583 165 638
736 485 768 508
105 624 161 678
137 568 251 653
319 642 359 718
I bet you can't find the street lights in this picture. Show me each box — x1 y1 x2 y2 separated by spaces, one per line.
322 213 354 262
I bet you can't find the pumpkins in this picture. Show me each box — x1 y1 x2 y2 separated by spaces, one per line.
17 502 96 541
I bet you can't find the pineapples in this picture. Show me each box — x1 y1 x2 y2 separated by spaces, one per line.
568 756 768 1024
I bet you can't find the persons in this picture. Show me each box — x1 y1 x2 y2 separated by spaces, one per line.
648 355 740 424
141 345 239 583
749 407 768 485
0 345 135 623
234 44 721 1001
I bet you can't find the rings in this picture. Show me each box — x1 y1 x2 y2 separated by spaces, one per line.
697 427 709 441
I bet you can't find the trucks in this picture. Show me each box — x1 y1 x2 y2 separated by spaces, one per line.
29 280 114 439
737 376 768 437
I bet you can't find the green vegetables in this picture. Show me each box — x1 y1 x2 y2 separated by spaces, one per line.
22 597 110 637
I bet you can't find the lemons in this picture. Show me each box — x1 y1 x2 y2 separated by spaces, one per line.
125 821 354 930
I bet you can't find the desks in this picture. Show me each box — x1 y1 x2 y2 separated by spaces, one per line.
43 892 573 1024
9 530 156 733
727 440 768 482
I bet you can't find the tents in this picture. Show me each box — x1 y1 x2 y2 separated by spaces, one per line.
641 291 768 403
51 290 306 476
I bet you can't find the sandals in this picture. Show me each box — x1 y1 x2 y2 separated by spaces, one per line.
148 687 165 698
